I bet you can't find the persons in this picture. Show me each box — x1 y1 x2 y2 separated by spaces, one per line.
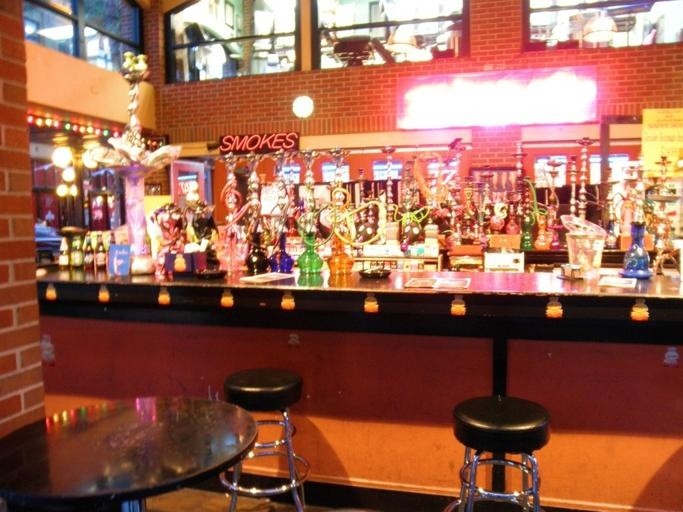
642 0 683 43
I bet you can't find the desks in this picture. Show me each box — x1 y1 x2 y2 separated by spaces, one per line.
0 398 260 512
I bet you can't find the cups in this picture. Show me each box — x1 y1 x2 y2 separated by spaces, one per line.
564 230 605 280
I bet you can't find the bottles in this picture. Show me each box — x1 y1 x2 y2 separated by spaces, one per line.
604 218 618 250
58 232 117 271
533 215 562 252
618 205 655 280
246 227 355 277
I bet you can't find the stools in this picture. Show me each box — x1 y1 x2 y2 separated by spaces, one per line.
443 394 551 512
219 368 313 510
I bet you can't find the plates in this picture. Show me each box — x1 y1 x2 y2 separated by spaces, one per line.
357 268 393 279
